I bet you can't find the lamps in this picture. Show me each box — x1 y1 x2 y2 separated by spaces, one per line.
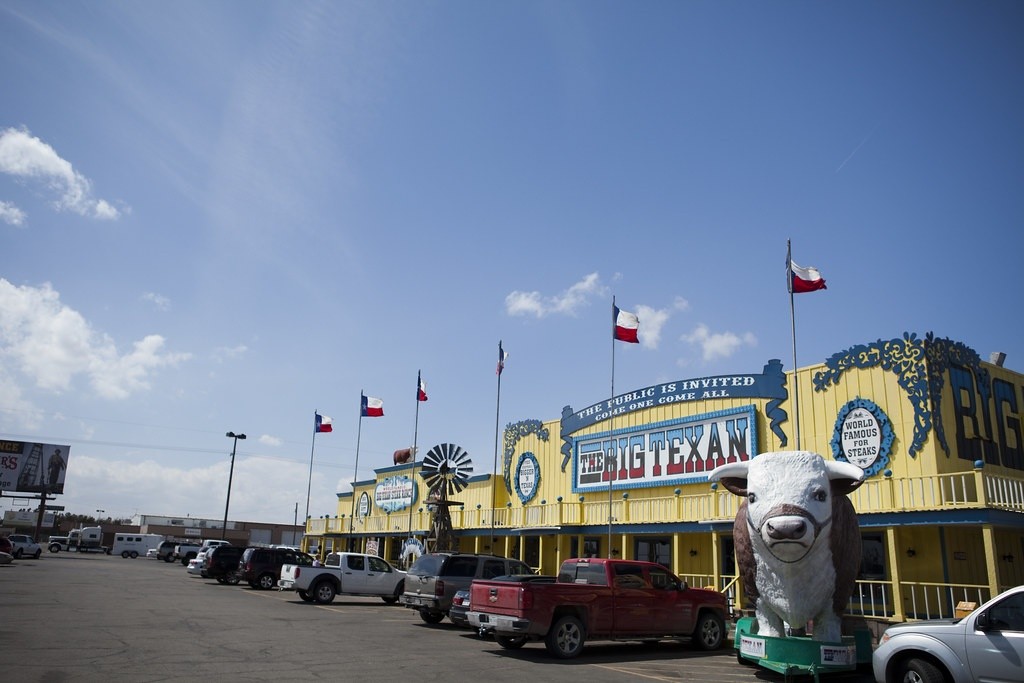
1003 551 1014 563
906 547 916 557
689 548 697 556
612 548 619 556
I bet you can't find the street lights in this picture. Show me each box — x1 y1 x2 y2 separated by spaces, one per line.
97 510 105 524
222 432 246 539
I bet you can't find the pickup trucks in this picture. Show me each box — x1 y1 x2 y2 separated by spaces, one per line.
277 553 408 604
173 539 234 566
465 558 731 659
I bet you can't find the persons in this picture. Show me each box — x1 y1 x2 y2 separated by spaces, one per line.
48 449 66 489
312 555 322 567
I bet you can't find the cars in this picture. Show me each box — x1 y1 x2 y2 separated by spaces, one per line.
449 574 557 628
187 552 206 575
873 585 1024 683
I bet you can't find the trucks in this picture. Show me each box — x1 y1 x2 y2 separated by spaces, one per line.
48 523 140 555
112 533 170 559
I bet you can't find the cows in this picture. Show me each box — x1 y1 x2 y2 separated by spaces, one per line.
708 451 864 643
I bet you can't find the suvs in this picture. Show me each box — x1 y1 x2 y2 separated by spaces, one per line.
399 551 537 623
0 536 42 564
235 546 325 590
157 540 201 563
200 544 249 585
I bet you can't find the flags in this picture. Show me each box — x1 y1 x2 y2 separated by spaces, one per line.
315 414 332 433
613 306 639 344
360 395 384 417
417 376 428 401
496 347 509 375
786 258 827 293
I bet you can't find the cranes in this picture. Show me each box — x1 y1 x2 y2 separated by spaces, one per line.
17 443 44 492
